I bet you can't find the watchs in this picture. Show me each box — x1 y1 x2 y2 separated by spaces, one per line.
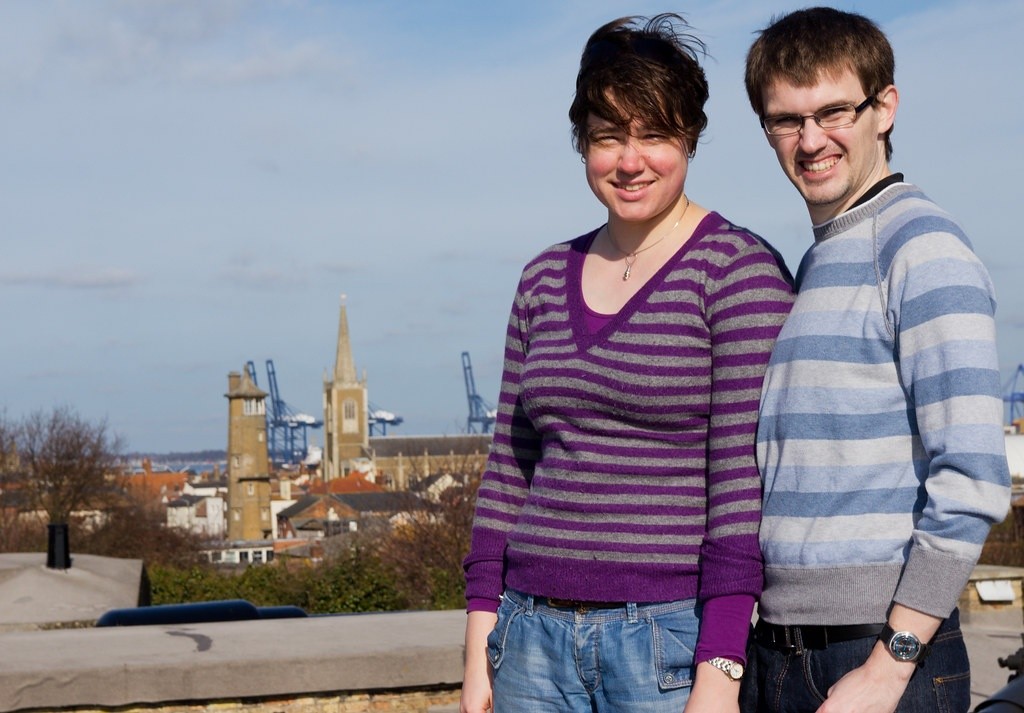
705 657 744 681
881 622 925 662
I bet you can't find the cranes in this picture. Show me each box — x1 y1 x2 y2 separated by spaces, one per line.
265 360 323 469
460 352 499 434
1001 364 1023 423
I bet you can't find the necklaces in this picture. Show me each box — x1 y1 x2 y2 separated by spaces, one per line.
606 192 689 281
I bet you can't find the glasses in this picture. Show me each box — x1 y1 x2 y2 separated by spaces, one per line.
760 92 876 136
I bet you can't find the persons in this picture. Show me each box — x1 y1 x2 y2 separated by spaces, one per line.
458 11 798 713
738 5 1011 713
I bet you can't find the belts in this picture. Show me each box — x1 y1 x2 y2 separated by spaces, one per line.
755 608 960 653
532 597 624 616
